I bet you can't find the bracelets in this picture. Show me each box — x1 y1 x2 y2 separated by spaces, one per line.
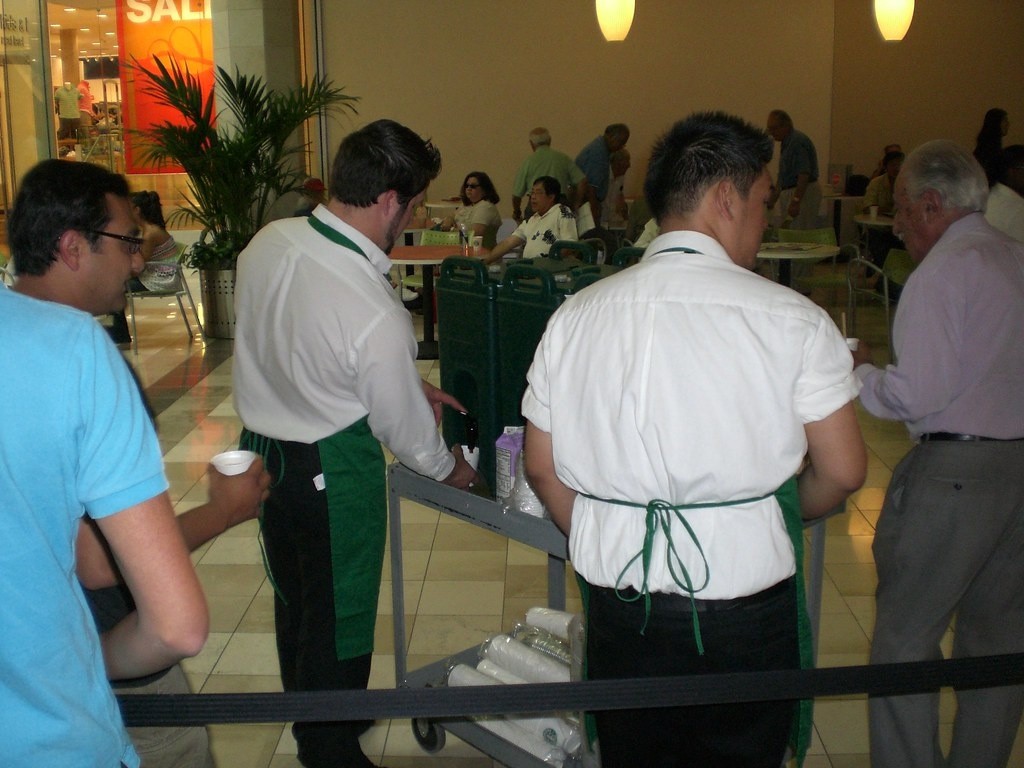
512 207 520 213
792 196 800 203
620 204 628 212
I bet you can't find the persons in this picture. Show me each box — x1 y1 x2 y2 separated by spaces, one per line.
473 176 578 264
416 171 503 315
762 110 823 296
850 139 1024 768
858 144 909 285
294 177 418 301
972 108 1024 242
520 111 866 768
233 119 480 768
0 160 270 768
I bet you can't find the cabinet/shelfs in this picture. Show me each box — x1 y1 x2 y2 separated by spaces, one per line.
383 462 848 768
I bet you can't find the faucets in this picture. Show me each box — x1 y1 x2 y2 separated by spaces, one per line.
460 412 480 450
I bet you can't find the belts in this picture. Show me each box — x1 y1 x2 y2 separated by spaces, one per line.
920 432 997 442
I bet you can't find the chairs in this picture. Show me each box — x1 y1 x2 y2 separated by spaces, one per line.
402 229 476 290
0 253 15 287
124 242 207 352
779 227 862 335
847 249 920 365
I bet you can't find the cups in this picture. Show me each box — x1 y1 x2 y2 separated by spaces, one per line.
495 424 546 519
472 236 483 251
450 445 479 488
870 206 878 219
212 450 257 476
445 606 587 767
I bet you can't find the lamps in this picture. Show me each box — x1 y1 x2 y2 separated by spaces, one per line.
596 0 635 42
874 0 916 41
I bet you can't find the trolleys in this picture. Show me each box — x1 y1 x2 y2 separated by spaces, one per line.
384 461 849 768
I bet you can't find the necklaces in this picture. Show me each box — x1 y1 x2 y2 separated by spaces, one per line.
468 195 487 205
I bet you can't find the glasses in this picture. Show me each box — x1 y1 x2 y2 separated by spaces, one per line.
530 192 545 195
465 184 481 189
56 227 145 256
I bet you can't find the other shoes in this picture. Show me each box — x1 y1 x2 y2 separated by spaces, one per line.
291 720 384 768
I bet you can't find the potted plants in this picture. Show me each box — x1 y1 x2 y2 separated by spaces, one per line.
115 52 365 342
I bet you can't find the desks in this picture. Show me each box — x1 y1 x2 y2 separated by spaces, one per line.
388 245 490 361
854 214 895 307
401 217 438 313
759 242 839 289
